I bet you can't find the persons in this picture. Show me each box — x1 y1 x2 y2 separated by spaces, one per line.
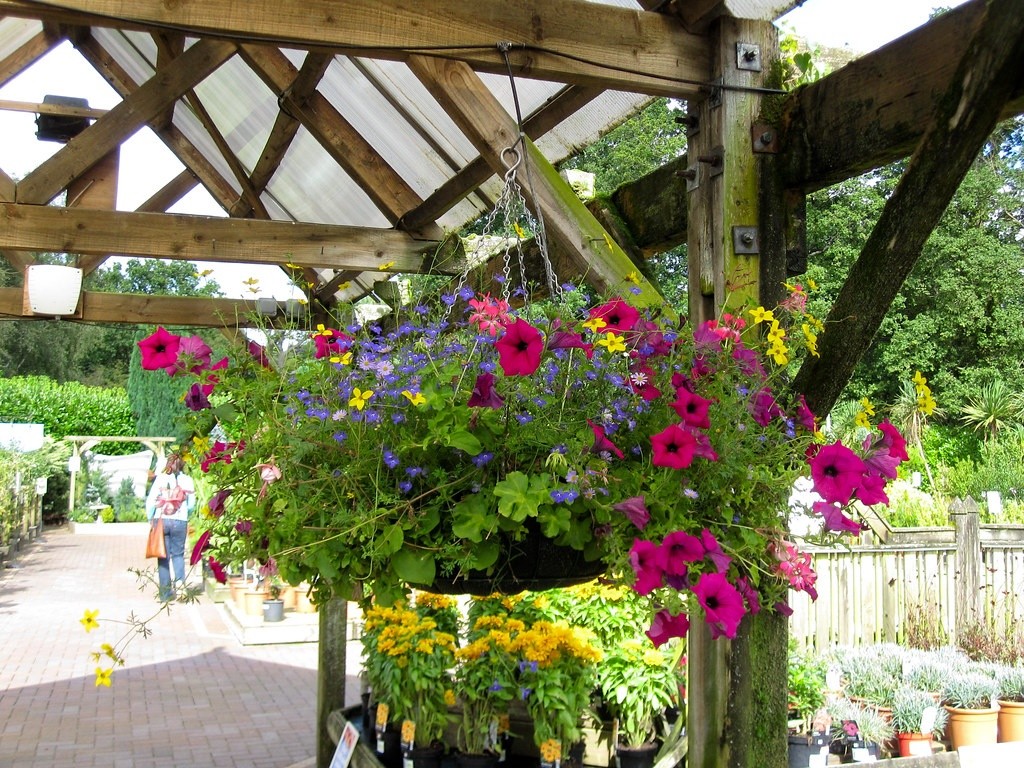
144 452 194 601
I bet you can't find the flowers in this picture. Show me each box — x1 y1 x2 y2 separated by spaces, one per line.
78 271 909 768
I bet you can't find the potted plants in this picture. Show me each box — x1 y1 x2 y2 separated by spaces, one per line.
838 640 1024 758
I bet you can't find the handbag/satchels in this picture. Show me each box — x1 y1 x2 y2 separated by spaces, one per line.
145 518 166 560
